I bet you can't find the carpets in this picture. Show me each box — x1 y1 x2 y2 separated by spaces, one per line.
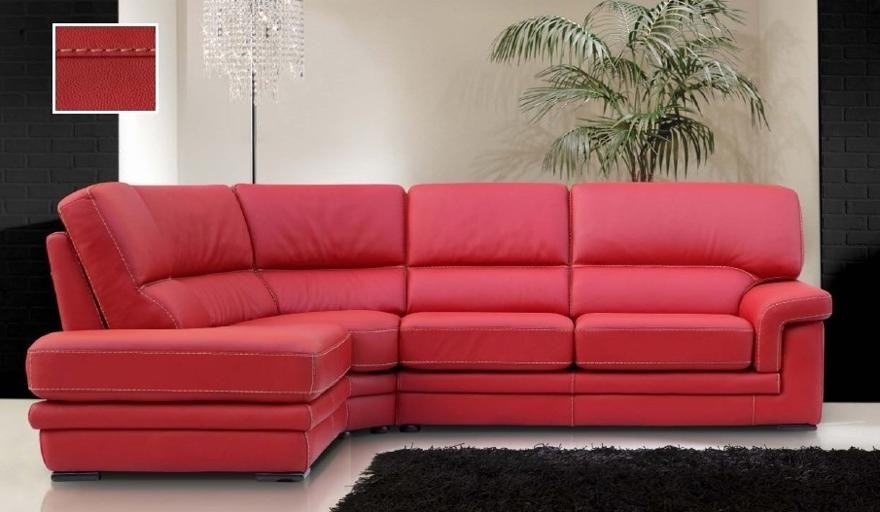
325 444 877 511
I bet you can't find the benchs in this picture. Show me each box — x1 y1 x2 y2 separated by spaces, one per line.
24 327 355 486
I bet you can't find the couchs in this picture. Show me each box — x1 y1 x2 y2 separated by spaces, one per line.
46 181 833 436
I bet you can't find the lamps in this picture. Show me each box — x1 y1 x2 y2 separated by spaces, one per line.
200 2 307 184
